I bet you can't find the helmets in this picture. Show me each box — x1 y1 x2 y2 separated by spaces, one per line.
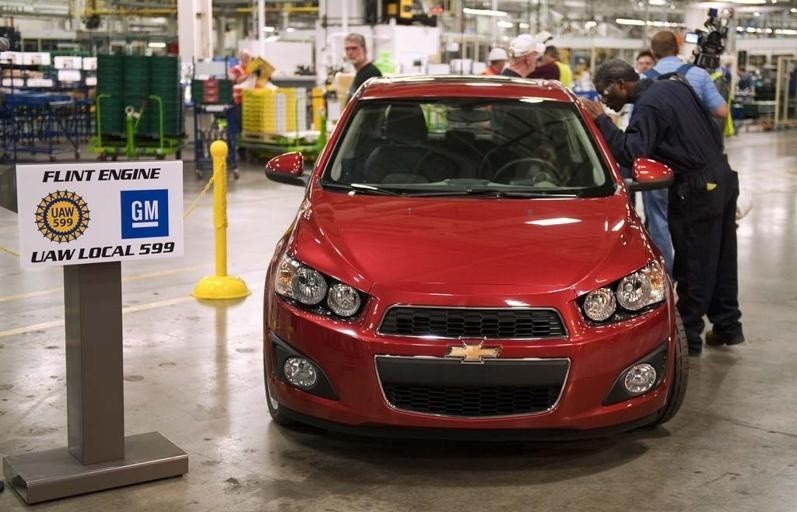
487 48 508 61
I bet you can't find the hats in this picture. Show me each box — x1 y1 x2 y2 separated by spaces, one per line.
508 30 555 58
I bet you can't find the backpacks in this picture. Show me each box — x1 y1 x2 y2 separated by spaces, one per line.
643 63 696 86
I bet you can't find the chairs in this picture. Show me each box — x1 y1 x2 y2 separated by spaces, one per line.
365 104 583 186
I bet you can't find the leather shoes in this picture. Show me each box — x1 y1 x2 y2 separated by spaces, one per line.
687 335 703 356
705 325 746 347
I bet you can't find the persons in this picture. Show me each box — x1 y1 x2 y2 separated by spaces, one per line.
480 29 571 113
626 32 730 281
579 57 745 355
340 33 382 109
620 50 657 213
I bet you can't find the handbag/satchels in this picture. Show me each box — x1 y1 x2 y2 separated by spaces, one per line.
733 186 755 220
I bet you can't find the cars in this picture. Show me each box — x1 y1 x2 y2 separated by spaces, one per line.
262 76 690 443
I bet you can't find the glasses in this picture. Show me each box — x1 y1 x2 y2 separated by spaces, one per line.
600 80 619 98
